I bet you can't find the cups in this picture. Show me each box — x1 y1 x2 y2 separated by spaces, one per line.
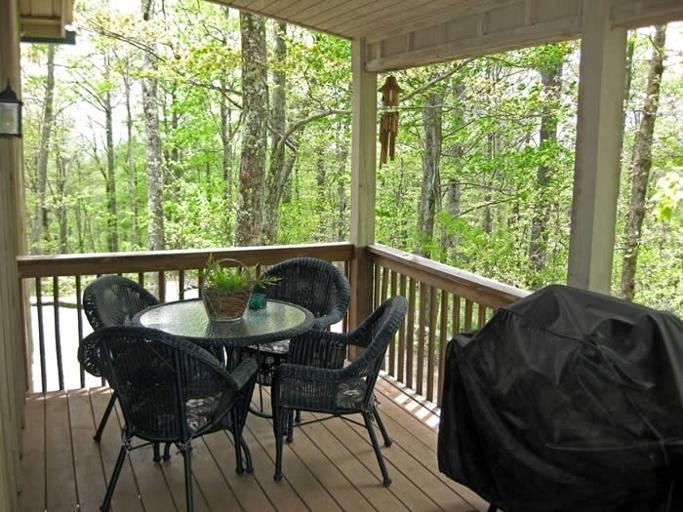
249 294 267 310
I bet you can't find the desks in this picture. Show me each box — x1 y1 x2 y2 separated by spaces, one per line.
128 292 317 477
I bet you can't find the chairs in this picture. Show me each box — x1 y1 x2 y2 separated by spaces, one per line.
228 258 352 446
81 275 169 444
272 294 406 487
79 325 263 512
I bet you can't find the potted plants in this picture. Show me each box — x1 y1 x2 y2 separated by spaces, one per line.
178 253 283 324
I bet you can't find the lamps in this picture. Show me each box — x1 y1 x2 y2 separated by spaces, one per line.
1 76 22 139
